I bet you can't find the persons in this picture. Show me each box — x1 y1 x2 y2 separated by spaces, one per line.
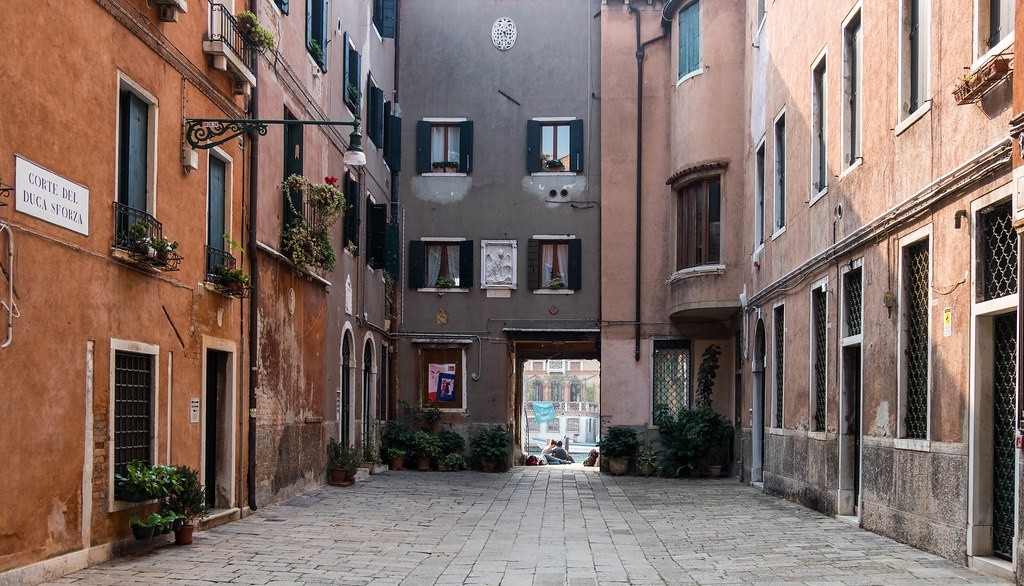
552 441 575 463
541 439 556 456
528 454 571 466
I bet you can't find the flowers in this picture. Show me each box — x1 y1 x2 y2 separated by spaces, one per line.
325 176 340 190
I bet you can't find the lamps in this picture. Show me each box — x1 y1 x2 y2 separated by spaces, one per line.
182 114 366 165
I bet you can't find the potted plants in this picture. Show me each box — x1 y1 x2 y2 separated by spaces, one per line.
436 458 446 471
707 446 726 477
161 513 173 535
150 237 178 263
387 447 407 468
432 161 459 173
236 10 261 36
545 159 565 172
634 436 663 476
116 459 183 502
595 427 641 475
686 461 700 477
327 437 347 482
409 430 438 469
169 511 183 531
220 265 248 292
152 512 164 537
129 515 155 540
469 424 513 472
173 466 208 544
